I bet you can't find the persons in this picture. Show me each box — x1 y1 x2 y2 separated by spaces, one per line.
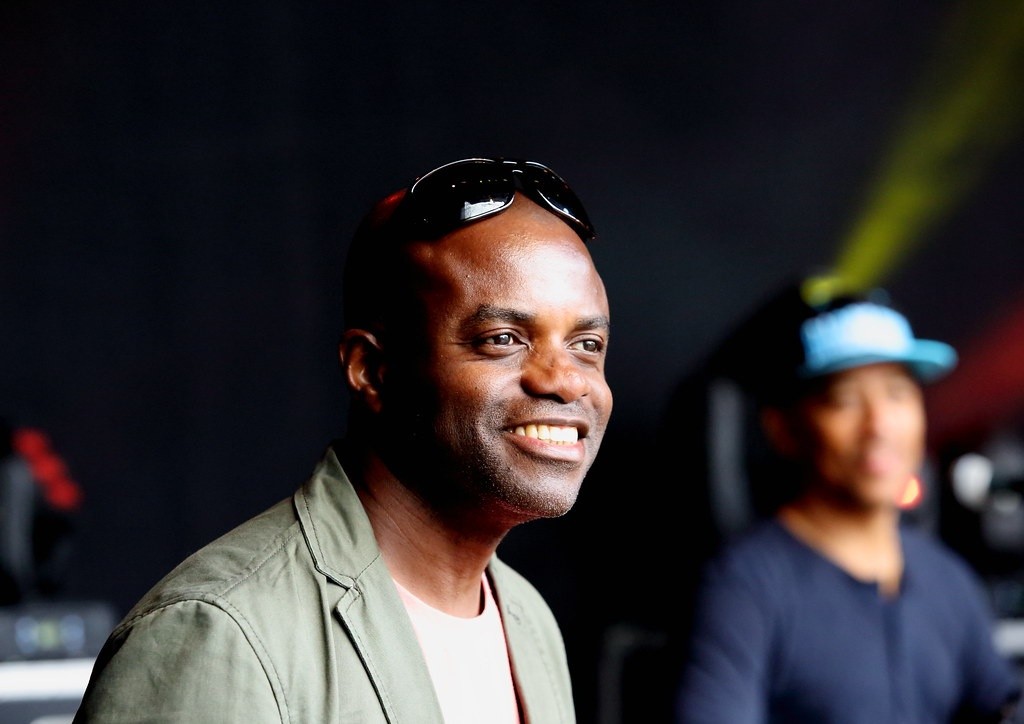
68 158 614 724
666 302 1024 724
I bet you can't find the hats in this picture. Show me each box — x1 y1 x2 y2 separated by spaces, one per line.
800 300 959 388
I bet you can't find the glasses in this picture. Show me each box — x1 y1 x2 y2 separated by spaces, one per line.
376 155 598 257
